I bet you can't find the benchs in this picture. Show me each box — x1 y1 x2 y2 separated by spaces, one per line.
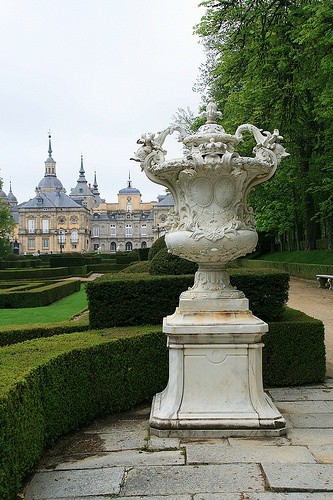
316 273 333 290
